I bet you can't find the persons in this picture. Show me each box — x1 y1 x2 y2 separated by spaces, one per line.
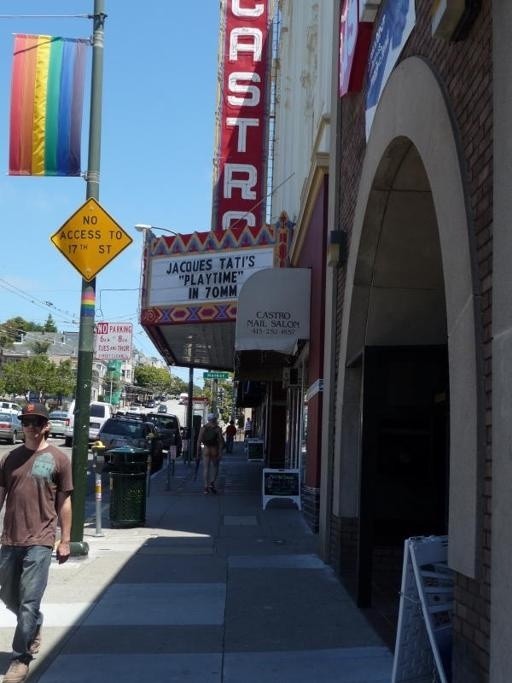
0 403 74 683
195 412 226 495
244 417 252 442
222 419 237 453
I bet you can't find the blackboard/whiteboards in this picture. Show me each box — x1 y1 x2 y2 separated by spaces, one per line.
247 441 265 461
410 536 452 683
263 468 301 498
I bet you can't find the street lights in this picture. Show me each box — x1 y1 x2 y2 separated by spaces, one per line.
135 221 195 459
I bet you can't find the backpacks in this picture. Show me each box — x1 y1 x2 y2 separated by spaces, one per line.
202 424 220 446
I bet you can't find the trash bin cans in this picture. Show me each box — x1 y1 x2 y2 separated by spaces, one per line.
104 445 151 529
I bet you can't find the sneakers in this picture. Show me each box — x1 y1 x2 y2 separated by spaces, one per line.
29 626 41 654
200 489 208 493
2 659 29 682
207 484 215 487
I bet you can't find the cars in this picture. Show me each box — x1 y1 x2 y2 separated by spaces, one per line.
0 392 189 475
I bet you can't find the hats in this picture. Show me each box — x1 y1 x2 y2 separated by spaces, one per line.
207 414 217 420
18 404 49 419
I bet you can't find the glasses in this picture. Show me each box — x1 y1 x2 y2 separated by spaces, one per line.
22 418 44 427
